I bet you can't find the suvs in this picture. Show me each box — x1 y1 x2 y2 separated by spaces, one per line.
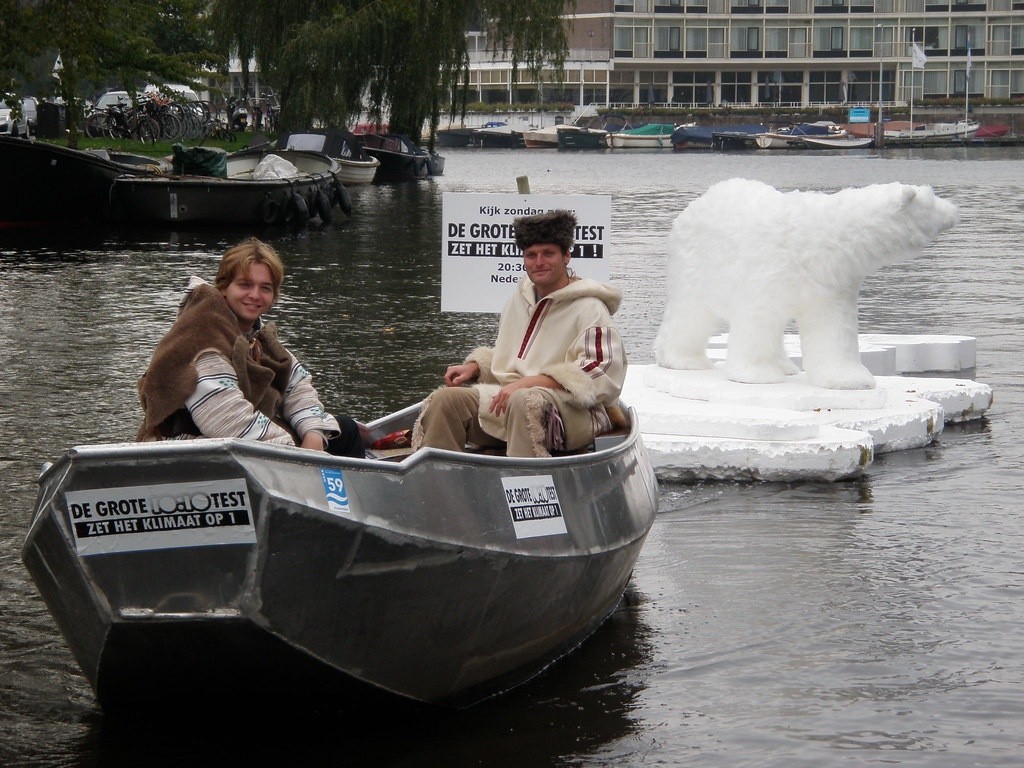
144 83 202 116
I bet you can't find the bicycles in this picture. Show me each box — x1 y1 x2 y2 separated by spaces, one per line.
0 86 283 145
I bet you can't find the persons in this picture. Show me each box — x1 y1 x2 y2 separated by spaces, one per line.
137 238 362 458
416 210 628 458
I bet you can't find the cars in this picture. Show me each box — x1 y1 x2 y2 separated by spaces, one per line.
84 90 151 134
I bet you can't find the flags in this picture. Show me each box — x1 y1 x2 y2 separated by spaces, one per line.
966 37 971 78
912 42 927 69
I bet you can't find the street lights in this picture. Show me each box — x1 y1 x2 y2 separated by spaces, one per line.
875 23 885 122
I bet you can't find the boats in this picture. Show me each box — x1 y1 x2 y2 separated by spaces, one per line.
344 130 430 182
19 392 660 726
264 127 381 186
0 130 175 236
436 124 1009 150
104 140 343 232
